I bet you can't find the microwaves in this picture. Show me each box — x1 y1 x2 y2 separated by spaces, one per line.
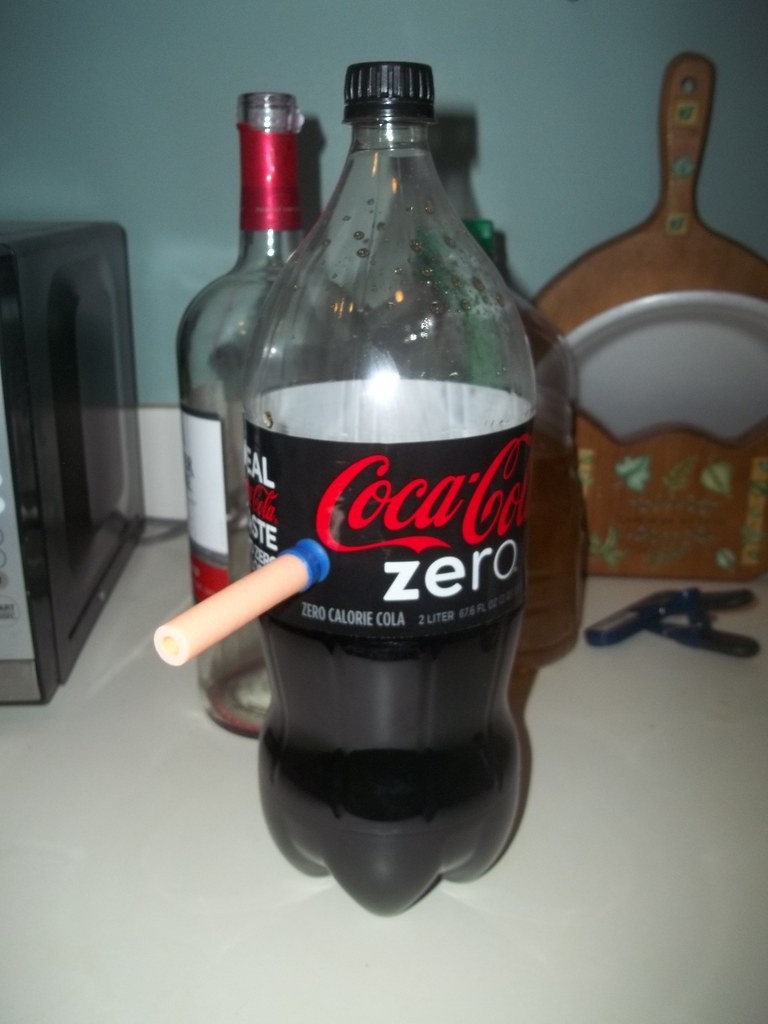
0 219 145 704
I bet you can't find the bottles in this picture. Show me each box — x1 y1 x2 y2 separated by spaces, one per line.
461 220 585 666
259 61 526 916
177 92 304 735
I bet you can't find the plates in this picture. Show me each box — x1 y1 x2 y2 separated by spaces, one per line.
539 290 768 440
